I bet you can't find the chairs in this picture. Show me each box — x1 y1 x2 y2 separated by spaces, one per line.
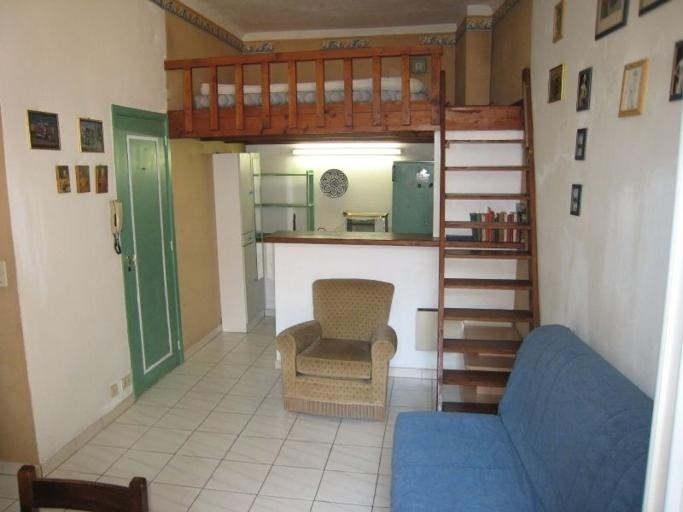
17 464 149 512
274 277 398 422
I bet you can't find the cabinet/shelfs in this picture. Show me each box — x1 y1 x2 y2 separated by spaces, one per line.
214 152 265 333
254 170 315 242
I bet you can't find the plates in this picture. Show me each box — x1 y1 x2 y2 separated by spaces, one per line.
318 169 347 199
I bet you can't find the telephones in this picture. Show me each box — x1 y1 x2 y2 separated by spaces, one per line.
110 201 123 234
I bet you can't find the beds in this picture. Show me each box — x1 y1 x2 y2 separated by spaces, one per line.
164 45 442 141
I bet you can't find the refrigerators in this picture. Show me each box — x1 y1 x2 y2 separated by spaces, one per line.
390 158 435 236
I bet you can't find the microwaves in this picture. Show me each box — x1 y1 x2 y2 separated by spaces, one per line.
344 217 386 232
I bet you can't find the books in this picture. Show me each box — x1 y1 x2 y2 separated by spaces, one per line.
470 203 527 243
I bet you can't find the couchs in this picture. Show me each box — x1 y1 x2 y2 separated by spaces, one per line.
390 324 654 512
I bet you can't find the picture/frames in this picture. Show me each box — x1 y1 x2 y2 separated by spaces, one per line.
27 109 109 194
547 1 683 216
